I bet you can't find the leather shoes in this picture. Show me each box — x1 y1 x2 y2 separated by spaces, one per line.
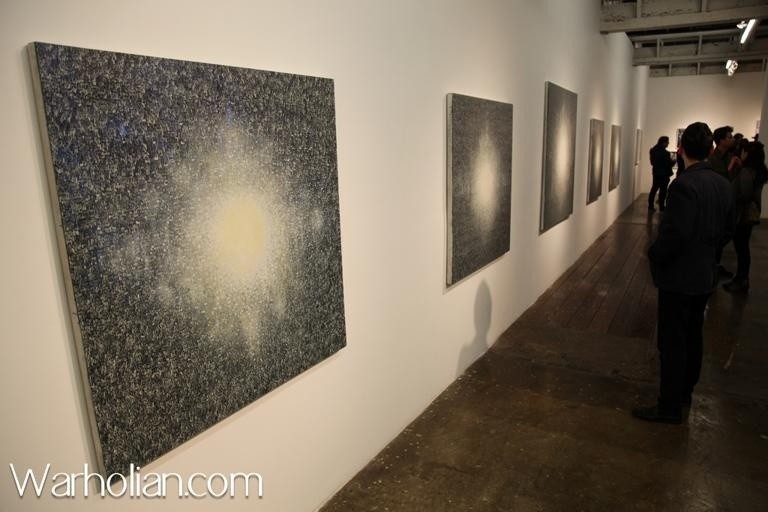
631 398 690 423
718 265 749 292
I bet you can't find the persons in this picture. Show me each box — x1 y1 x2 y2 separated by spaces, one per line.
631 122 738 425
676 125 768 294
648 136 677 211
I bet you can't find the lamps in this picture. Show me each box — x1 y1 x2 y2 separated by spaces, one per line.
737 19 756 44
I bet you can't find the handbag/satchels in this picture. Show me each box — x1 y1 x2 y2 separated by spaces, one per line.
736 203 760 225
